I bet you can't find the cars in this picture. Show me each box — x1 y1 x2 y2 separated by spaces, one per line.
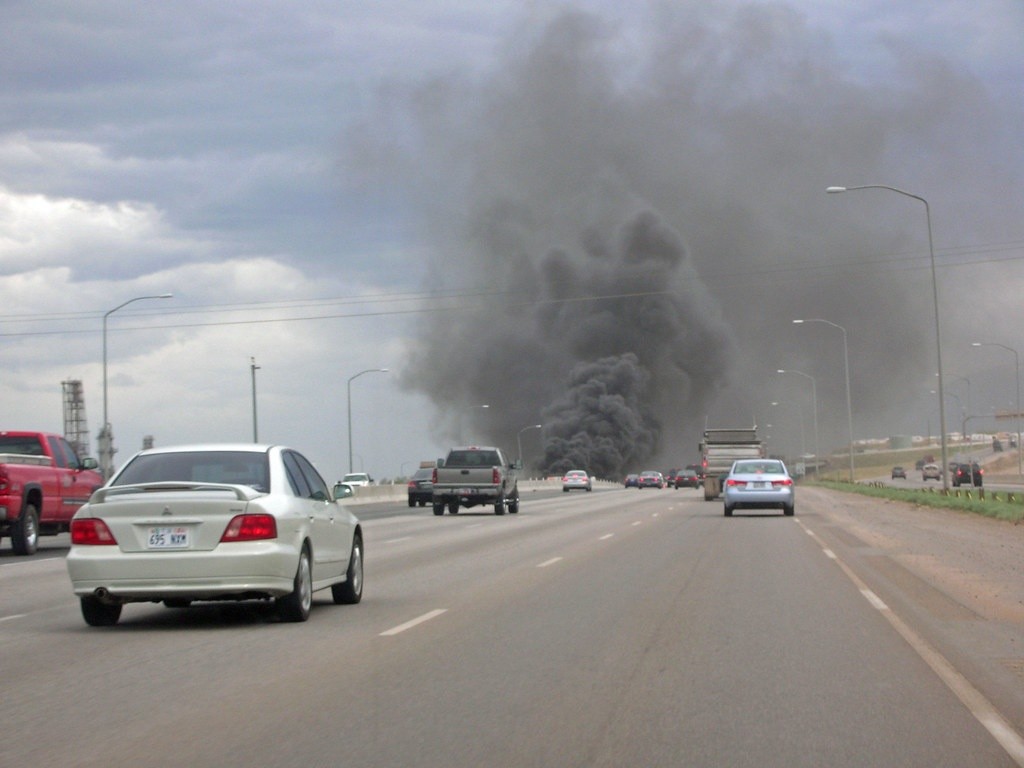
65 444 365 626
950 463 984 487
891 466 907 480
408 468 436 508
340 472 375 489
666 468 683 488
625 473 639 488
562 470 593 492
722 458 797 516
638 471 664 489
915 454 942 482
674 470 701 490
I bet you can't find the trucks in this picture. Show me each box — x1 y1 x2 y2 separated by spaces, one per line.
697 425 764 502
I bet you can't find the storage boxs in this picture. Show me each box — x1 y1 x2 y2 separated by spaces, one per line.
0 453 53 466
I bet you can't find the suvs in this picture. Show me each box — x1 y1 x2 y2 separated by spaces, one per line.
687 464 703 483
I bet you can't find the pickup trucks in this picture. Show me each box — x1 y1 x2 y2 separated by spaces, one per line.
431 446 520 517
0 429 107 557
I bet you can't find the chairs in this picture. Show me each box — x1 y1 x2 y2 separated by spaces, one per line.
451 453 469 466
157 461 186 482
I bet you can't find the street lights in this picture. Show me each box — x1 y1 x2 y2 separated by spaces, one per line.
823 183 952 493
100 293 175 482
517 424 543 469
346 367 389 472
972 342 1022 475
458 404 491 446
776 369 820 478
250 355 261 443
934 372 973 414
772 402 805 464
791 320 855 484
